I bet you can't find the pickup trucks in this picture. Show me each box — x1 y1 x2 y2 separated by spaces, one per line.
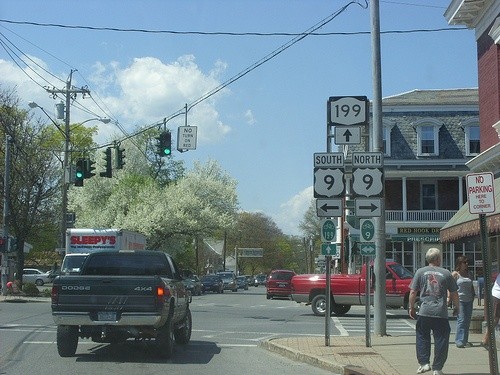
288 261 420 320
51 249 194 357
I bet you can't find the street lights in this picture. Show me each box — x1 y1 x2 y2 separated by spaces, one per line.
29 102 111 267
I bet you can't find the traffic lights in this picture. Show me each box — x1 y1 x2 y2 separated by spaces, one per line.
75 159 84 186
115 145 125 169
100 147 111 178
155 132 171 157
0 237 6 252
84 160 96 179
10 238 18 251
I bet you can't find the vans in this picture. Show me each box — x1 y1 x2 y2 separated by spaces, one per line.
267 269 297 300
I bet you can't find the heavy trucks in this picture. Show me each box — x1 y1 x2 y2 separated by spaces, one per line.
50 228 146 276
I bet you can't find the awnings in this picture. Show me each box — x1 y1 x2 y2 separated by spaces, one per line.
439 176 500 245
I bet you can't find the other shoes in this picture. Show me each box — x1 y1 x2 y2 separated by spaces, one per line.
465 342 473 347
480 342 488 350
416 364 431 374
456 342 464 347
433 370 445 375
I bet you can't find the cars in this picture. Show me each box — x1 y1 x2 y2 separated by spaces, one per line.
13 268 52 286
182 271 267 296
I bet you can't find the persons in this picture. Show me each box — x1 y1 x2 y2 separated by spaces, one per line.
482 273 500 350
447 257 476 348
409 248 458 375
